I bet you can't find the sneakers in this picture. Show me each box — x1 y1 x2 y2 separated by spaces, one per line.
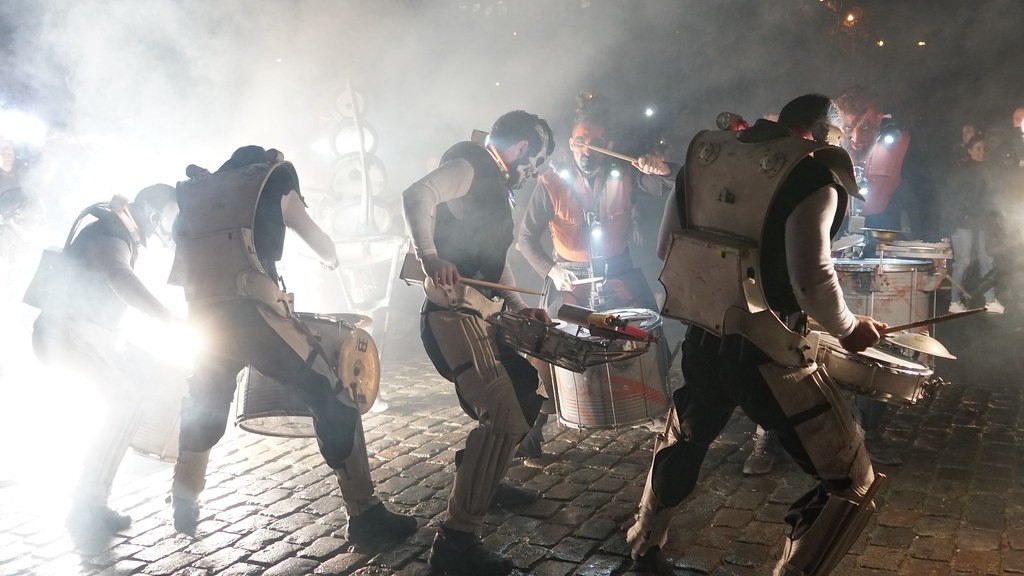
949 301 967 313
743 434 785 474
985 298 1007 313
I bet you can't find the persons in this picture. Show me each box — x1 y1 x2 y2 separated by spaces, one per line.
742 88 938 475
400 109 555 574
31 183 185 542
623 92 890 575
167 144 418 543
930 106 1024 314
516 92 683 462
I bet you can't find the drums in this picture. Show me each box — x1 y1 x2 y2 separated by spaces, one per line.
825 257 934 362
547 306 671 430
233 310 360 440
495 311 592 374
813 330 934 406
875 239 954 316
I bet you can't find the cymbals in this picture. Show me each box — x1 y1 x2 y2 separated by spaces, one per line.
886 332 957 361
329 313 373 327
860 228 906 241
340 327 381 413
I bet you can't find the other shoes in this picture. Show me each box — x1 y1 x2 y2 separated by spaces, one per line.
172 496 204 532
70 502 131 532
428 523 514 576
455 449 543 506
345 496 417 544
